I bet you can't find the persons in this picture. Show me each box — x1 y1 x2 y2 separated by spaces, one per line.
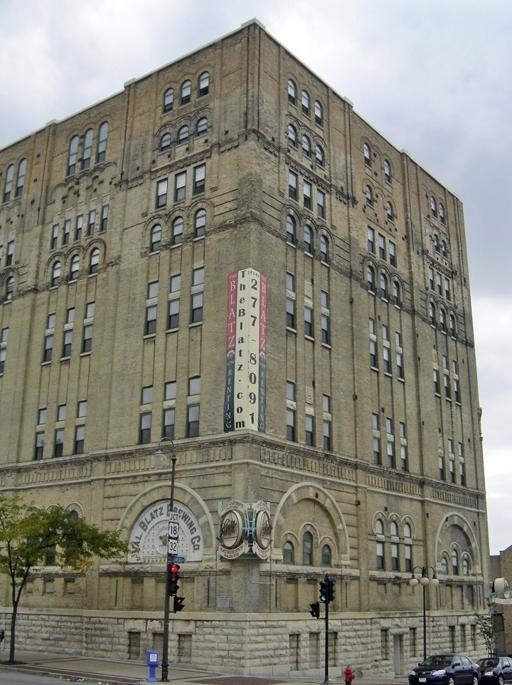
344 664 352 685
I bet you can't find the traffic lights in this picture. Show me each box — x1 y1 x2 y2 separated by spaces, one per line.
319 579 335 602
168 562 180 596
174 597 184 612
309 602 318 617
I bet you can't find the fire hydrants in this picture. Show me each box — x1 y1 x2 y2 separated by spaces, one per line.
344 665 355 685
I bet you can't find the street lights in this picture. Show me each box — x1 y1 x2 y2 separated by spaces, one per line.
150 438 177 682
409 566 439 662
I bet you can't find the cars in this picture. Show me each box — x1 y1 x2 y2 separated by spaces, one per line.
409 654 483 685
476 657 512 684
